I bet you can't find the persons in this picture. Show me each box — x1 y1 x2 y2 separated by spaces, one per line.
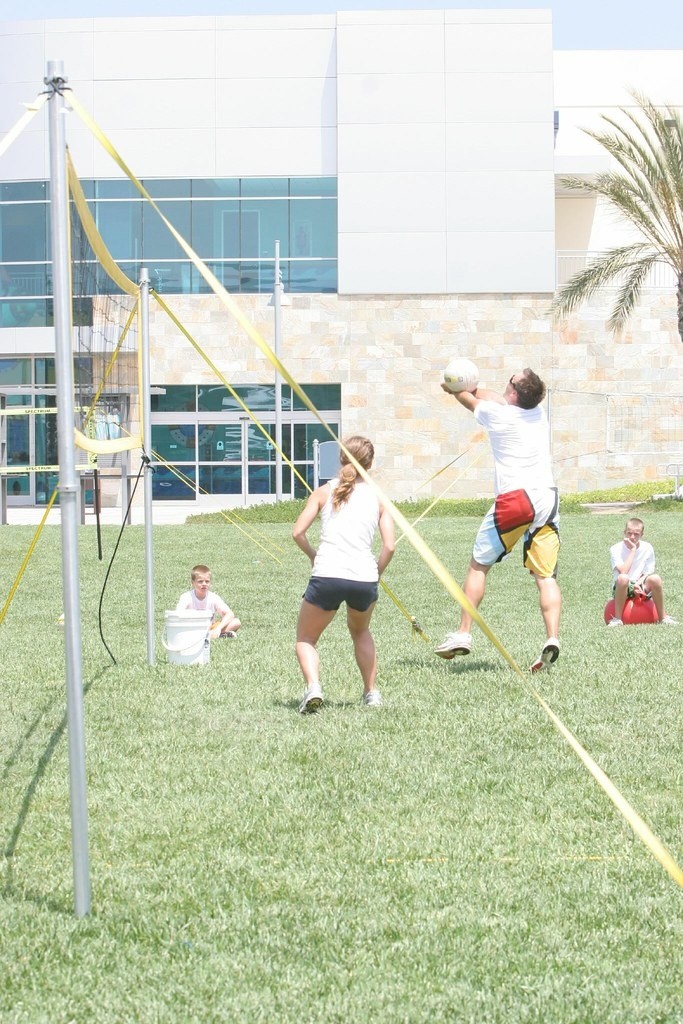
174 566 242 639
433 368 562 674
606 518 678 627
292 436 396 715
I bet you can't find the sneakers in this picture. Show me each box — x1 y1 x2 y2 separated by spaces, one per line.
360 691 381 706
529 637 560 671
434 631 471 660
300 684 324 714
607 614 623 628
657 615 678 625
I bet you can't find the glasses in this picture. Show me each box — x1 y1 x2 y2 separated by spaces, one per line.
510 374 518 391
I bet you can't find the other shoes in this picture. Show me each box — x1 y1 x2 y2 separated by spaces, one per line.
220 631 237 639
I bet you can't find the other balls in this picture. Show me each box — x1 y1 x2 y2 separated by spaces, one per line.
605 593 664 626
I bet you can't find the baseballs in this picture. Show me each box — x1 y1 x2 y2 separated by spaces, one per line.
442 357 480 392
